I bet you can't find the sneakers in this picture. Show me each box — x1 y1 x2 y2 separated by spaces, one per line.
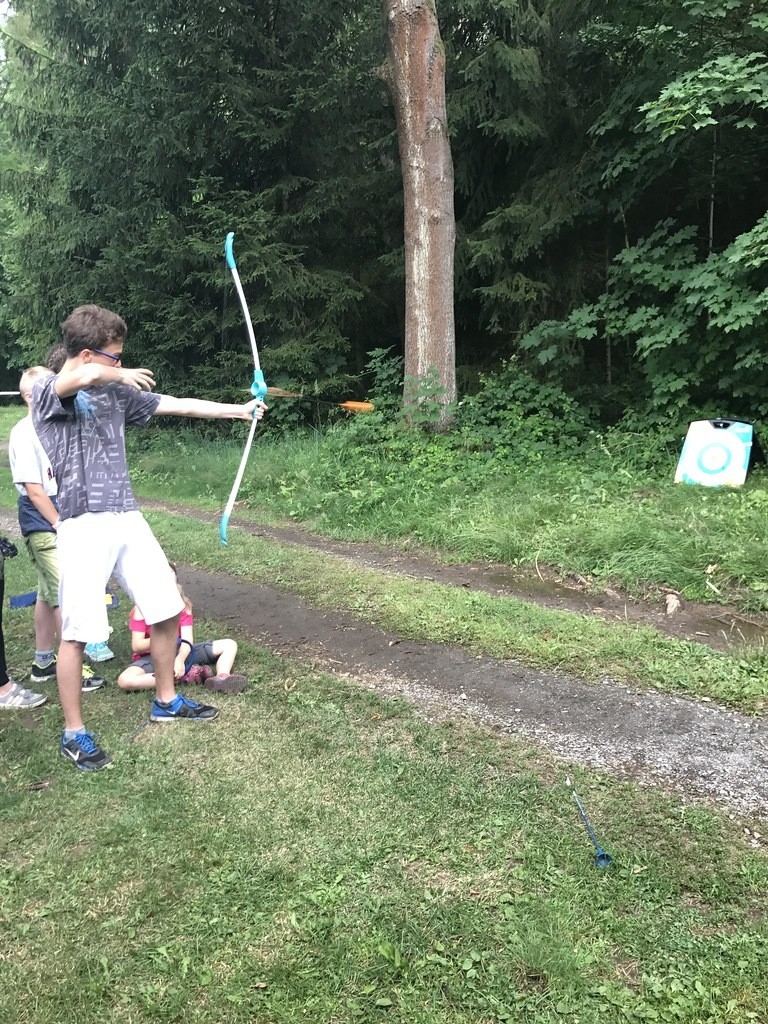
60 729 111 771
82 664 107 692
0 683 47 710
205 673 247 694
150 694 219 722
30 654 57 683
181 665 213 684
84 641 116 662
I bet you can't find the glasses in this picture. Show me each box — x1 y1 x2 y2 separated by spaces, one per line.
93 349 121 364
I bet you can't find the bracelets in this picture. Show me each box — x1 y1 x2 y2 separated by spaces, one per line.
51 515 62 529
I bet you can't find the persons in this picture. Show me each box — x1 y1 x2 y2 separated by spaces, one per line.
116 562 249 694
45 345 117 663
31 302 269 774
8 365 108 693
0 539 48 710
